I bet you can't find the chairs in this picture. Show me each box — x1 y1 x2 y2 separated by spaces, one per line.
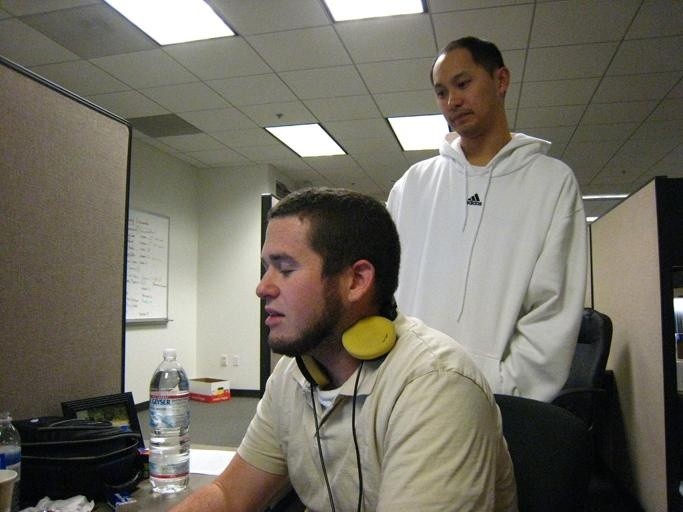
491 392 597 512
554 304 613 469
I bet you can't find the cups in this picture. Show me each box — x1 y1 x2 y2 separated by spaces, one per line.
0 469 17 512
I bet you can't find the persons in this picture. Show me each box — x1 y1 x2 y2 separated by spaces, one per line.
381 37 591 406
165 187 521 511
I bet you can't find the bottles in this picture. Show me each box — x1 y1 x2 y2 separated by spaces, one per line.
0 410 21 512
146 348 192 495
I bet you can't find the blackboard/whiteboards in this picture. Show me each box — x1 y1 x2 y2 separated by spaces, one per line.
124 206 170 323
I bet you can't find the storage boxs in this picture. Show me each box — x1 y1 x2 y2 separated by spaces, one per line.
187 378 230 403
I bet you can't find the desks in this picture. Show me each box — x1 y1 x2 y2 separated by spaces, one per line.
70 437 293 512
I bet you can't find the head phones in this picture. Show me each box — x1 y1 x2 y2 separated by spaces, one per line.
295 297 399 389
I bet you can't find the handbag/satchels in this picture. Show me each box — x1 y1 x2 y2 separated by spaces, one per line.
12 416 142 500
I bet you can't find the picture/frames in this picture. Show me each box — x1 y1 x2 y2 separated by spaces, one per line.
59 391 146 450
